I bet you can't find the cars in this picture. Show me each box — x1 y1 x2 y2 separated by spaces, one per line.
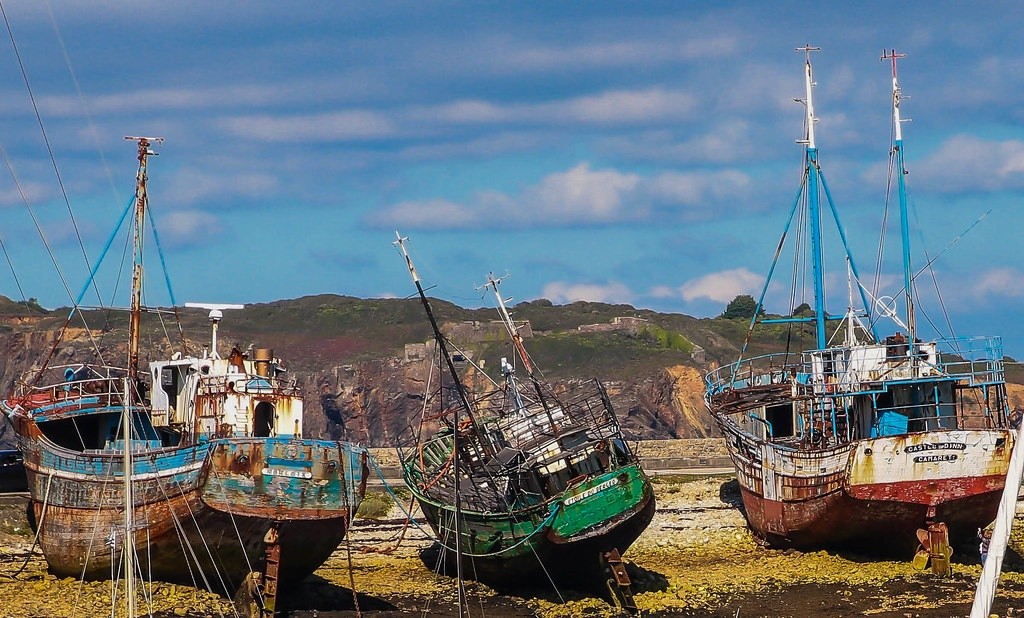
0 449 29 492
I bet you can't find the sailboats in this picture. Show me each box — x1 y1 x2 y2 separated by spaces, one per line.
702 42 1022 573
0 136 369 618
393 231 656 614
